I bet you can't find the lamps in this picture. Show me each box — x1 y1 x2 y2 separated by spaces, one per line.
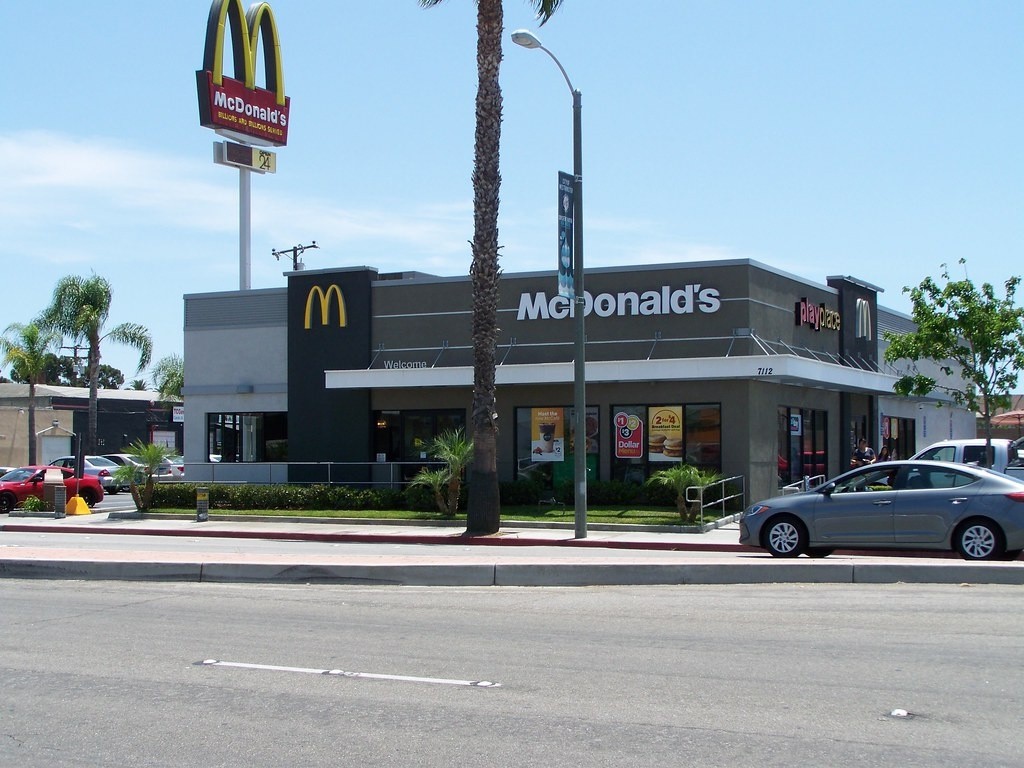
377 416 388 429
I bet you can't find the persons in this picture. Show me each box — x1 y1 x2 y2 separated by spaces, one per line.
867 472 897 491
850 438 876 483
876 445 890 463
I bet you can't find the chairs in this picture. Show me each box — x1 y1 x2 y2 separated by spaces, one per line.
909 475 934 488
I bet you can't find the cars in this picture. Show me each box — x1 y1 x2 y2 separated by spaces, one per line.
0 466 36 480
102 453 184 486
0 465 104 515
47 455 138 495
738 460 1024 562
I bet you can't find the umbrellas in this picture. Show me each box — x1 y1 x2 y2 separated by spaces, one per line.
990 409 1024 438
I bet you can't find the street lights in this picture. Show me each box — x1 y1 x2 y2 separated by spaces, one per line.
511 28 588 539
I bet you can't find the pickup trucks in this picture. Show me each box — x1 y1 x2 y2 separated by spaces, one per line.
873 437 1024 489
